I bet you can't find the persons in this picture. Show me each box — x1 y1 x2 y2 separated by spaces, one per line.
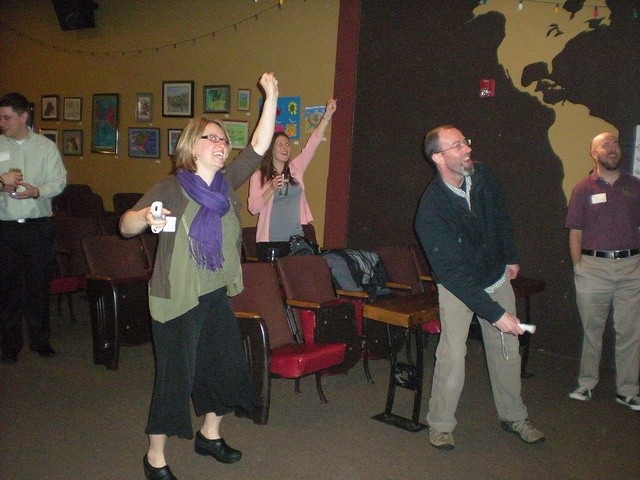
564 131 639 411
247 98 333 262
119 71 280 476
0 92 67 363
411 125 547 451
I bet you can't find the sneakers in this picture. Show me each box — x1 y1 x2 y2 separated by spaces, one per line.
616 394 640 411
428 427 455 451
569 387 594 401
501 420 548 444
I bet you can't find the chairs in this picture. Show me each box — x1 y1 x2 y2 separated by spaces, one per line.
81 236 151 369
49 218 99 324
51 183 93 219
331 250 412 383
228 260 346 425
373 245 430 364
98 216 125 237
278 255 374 401
410 243 438 292
142 231 159 266
112 193 143 214
240 222 317 261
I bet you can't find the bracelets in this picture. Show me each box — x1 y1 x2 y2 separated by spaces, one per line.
323 117 331 122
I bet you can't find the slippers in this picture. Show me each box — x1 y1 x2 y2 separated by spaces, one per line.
201 134 229 146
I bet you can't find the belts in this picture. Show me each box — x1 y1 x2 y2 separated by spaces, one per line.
581 248 640 258
0 216 52 224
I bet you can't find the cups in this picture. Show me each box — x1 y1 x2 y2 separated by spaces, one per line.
277 172 290 196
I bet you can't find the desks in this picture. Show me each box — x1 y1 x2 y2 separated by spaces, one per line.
363 276 545 432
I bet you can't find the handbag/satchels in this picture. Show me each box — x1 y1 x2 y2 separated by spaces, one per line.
287 234 319 255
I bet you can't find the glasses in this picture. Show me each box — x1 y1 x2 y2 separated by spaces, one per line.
434 138 472 154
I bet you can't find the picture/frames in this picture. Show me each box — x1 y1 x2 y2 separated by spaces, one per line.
203 85 231 112
135 92 152 121
168 129 183 156
127 127 160 158
41 94 59 120
25 102 34 129
38 128 59 146
62 129 83 156
162 80 194 118
221 120 250 150
63 97 82 121
236 89 251 111
91 93 118 154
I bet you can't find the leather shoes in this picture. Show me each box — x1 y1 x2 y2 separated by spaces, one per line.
142 454 177 480
194 430 241 464
0 348 18 363
29 342 56 358
